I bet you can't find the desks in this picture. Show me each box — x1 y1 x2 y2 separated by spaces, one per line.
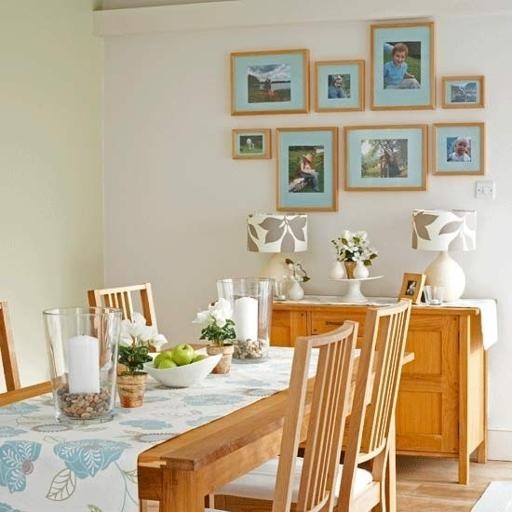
216 294 495 483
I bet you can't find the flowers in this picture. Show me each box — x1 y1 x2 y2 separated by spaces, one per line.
331 226 379 268
281 256 311 286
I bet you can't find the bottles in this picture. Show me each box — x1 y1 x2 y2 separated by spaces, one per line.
423 251 467 303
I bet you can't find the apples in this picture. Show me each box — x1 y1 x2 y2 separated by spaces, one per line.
154 343 208 368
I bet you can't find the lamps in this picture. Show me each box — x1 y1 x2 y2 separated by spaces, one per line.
411 206 479 303
245 211 310 298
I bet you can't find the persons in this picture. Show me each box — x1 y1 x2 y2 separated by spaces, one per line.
263 81 268 101
380 150 389 177
267 78 273 101
298 151 321 192
447 138 470 163
247 138 252 151
384 44 421 90
328 75 348 99
385 155 400 177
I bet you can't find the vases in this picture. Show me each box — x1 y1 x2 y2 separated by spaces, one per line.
350 258 371 279
287 278 306 302
344 257 355 279
331 257 346 281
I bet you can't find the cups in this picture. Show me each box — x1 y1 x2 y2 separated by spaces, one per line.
273 278 288 301
423 285 443 304
217 277 272 361
44 307 121 421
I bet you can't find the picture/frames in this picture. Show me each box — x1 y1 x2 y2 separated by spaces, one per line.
396 272 428 305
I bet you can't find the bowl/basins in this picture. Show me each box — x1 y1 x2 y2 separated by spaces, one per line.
144 354 223 387
